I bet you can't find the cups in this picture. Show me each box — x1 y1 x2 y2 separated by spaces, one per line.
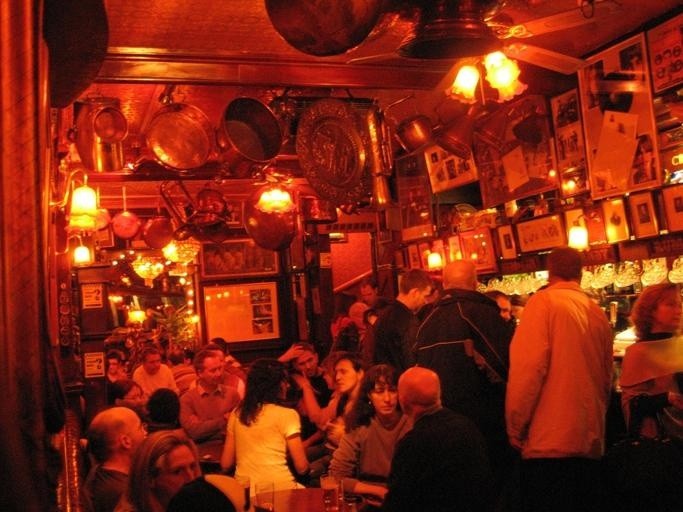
485 257 683 293
234 475 250 510
320 468 344 511
253 481 275 510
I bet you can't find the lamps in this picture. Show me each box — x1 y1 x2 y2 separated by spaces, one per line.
65 234 91 268
250 181 297 216
65 170 110 232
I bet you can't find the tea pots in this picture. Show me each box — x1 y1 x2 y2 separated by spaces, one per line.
383 91 445 152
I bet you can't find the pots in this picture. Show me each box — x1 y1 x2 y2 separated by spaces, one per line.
90 181 230 251
67 87 283 182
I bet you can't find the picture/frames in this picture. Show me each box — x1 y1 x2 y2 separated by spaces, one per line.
200 280 284 346
380 96 549 274
548 11 682 273
198 235 281 281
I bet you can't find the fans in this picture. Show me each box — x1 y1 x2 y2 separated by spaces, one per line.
345 0 620 99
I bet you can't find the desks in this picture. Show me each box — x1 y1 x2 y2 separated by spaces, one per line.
192 438 325 475
251 487 380 512
